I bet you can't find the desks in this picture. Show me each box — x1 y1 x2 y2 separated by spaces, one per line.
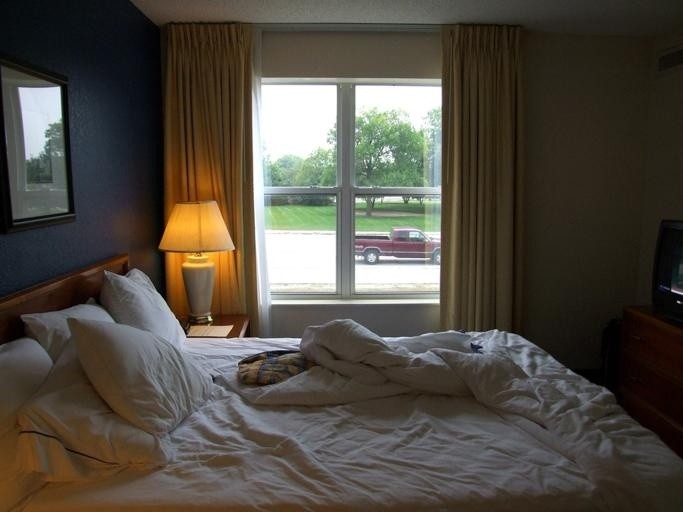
617 304 683 458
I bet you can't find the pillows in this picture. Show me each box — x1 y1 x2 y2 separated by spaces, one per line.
0 336 54 510
65 316 222 441
17 335 179 490
100 265 190 349
19 295 116 362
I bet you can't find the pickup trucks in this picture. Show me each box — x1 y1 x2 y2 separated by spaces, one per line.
355 227 441 266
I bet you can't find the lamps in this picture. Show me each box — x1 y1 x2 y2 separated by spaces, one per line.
157 199 236 325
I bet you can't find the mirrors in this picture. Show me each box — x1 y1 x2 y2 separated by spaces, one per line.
0 50 77 236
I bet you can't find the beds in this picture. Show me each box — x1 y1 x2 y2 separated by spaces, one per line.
0 251 683 511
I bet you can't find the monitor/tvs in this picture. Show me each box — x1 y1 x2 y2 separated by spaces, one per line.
652 219 683 322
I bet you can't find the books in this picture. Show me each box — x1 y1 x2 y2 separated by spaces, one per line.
187 324 236 338
